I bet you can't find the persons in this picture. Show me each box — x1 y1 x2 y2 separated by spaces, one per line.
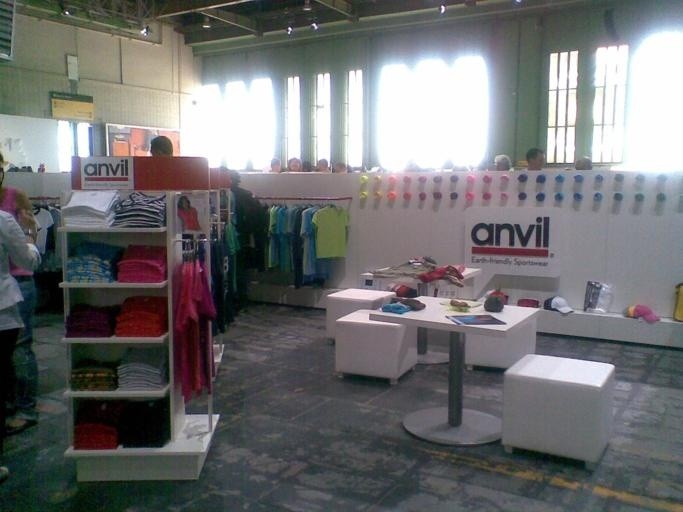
494 155 515 172
268 157 349 175
521 148 547 171
175 196 200 232
0 208 41 483
0 152 42 435
574 157 592 170
150 135 172 158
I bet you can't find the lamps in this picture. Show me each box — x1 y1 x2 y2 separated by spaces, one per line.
202 14 210 27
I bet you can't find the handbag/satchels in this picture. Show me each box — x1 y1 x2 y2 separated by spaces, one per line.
582 278 615 315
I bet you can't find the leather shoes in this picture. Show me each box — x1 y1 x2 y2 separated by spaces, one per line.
3 413 38 435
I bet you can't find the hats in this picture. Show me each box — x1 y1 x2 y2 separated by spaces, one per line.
543 294 575 315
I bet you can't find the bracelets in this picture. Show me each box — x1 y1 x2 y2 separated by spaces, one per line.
22 228 34 241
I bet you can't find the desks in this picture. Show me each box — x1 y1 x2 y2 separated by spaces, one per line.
369 295 541 447
360 267 482 364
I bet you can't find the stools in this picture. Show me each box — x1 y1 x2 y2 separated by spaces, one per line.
465 317 537 371
501 354 615 471
335 309 418 386
326 288 397 345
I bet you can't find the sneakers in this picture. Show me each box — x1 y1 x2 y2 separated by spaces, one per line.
0 466 9 479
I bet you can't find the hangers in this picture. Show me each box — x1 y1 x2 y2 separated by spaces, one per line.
264 197 342 211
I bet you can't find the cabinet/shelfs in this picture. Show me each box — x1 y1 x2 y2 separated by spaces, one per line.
56 157 232 481
